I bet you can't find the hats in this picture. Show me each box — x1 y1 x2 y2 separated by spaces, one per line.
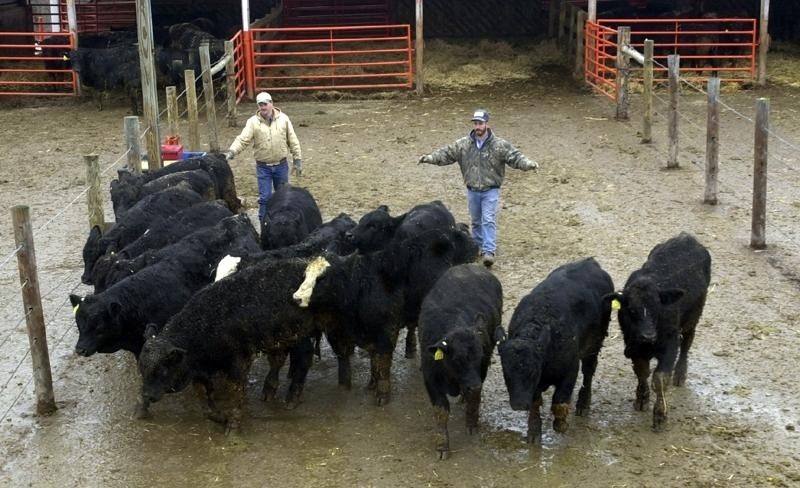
257 92 272 104
471 111 489 122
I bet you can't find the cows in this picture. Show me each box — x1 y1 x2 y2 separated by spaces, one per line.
418 235 711 461
70 155 482 430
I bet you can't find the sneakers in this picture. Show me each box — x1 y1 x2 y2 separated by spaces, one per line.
483 255 494 265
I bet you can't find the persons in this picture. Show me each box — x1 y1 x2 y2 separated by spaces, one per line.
416 108 539 265
222 92 302 223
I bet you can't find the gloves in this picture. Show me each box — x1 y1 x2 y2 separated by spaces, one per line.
526 161 539 174
291 159 302 176
417 155 433 165
223 152 234 160
